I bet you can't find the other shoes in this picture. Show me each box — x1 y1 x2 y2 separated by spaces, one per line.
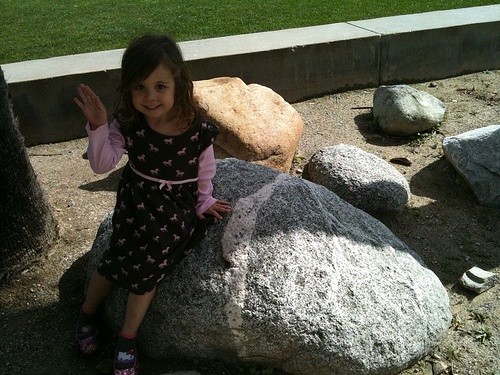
76 322 97 352
113 348 137 375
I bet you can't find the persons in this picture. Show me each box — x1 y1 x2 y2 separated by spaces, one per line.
74 34 233 375
